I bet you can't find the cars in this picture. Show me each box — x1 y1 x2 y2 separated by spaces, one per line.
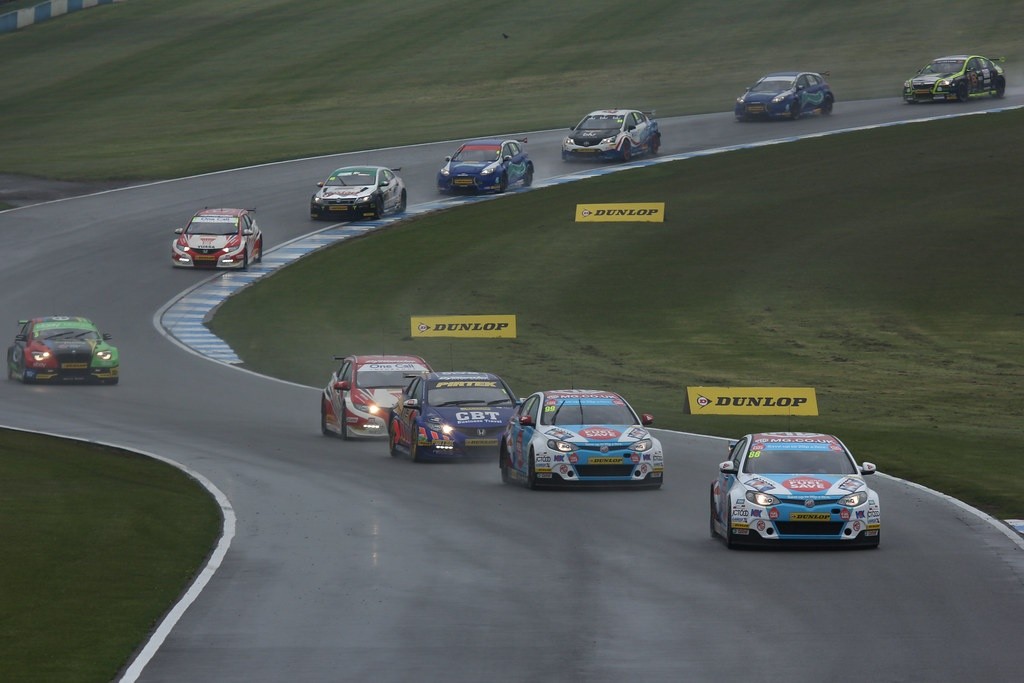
708 429 884 552
321 352 436 440
170 204 263 271
738 69 837 123
502 388 665 493
436 136 537 197
8 315 118 388
902 54 1005 104
389 367 523 462
560 109 659 163
310 165 407 222
